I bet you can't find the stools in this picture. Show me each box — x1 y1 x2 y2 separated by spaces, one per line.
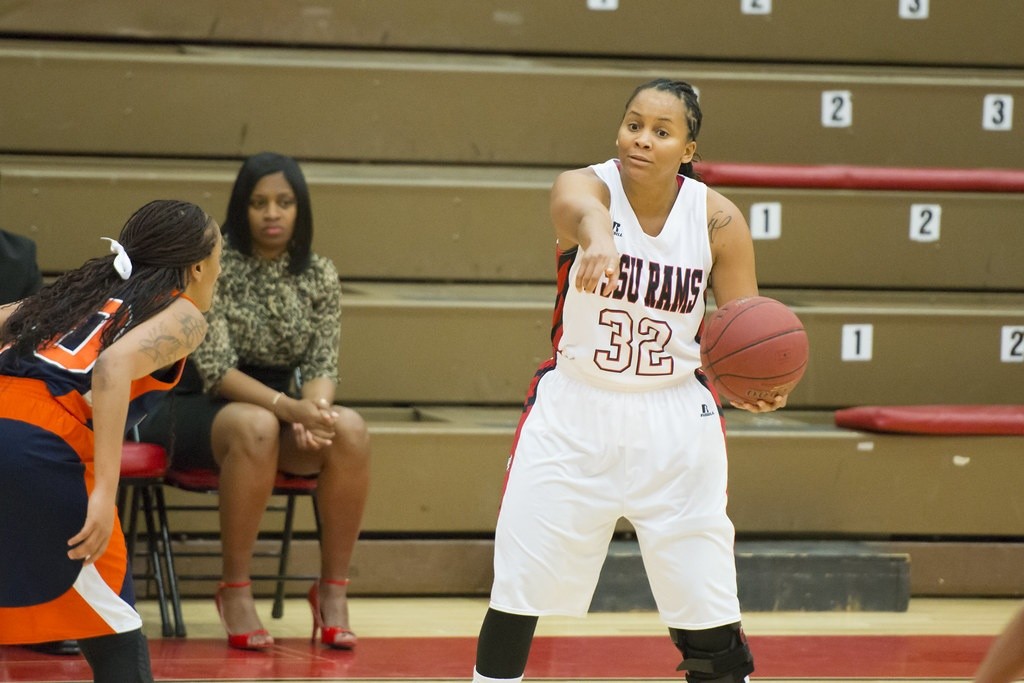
114 441 189 641
125 465 325 639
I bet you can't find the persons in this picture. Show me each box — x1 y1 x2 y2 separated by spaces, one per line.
0 227 84 657
123 151 371 650
472 79 788 683
0 200 225 683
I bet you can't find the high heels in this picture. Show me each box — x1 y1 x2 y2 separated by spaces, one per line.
308 576 357 647
216 581 274 649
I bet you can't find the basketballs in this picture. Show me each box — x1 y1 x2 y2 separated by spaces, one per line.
700 297 809 405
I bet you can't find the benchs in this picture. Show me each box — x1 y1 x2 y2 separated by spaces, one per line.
834 403 1023 598
691 160 1024 311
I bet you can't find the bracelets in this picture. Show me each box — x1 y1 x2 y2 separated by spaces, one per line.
271 391 286 419
